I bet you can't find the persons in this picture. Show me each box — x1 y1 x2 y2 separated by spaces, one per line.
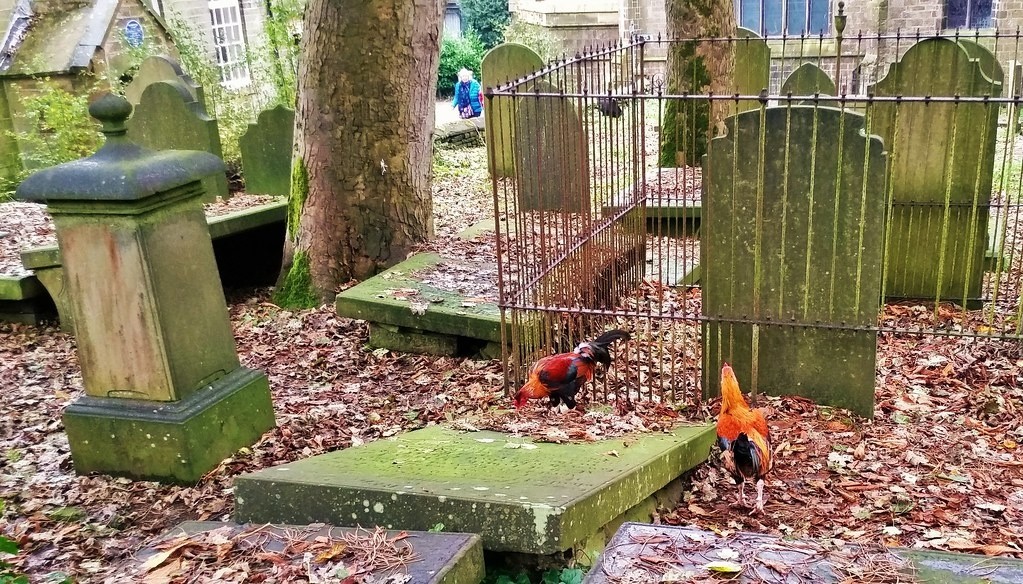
450 68 482 119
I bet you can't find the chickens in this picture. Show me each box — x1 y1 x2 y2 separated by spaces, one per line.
716 361 773 518
512 328 631 417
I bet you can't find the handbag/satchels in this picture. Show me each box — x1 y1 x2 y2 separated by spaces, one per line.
461 104 474 119
